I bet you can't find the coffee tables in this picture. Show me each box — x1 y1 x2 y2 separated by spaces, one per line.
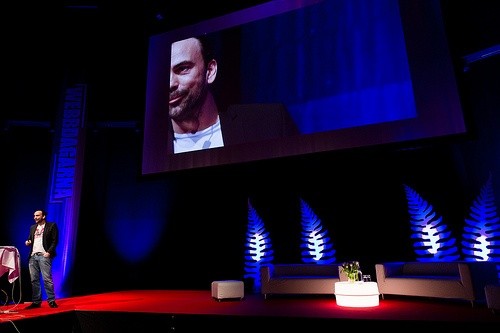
335 281 380 307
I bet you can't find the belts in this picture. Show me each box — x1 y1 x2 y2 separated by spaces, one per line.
36 252 44 256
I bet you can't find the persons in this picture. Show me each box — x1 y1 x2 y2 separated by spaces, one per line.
169 38 302 155
25 209 59 311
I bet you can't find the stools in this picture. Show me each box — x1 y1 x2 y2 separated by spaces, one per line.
211 280 244 302
485 284 500 313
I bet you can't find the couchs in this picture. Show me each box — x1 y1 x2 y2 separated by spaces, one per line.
261 263 349 300
375 261 474 307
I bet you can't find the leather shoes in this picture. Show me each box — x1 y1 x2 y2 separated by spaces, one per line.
25 303 40 309
49 301 57 308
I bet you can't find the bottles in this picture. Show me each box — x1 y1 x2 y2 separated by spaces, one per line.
363 275 371 282
345 262 358 281
